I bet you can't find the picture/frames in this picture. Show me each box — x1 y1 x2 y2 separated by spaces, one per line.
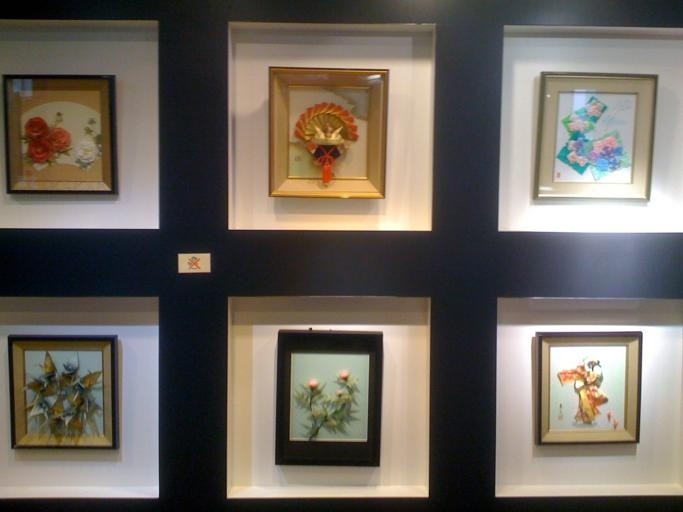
274 327 386 471
265 67 392 203
2 72 122 199
530 69 659 204
7 332 125 454
532 330 645 447
